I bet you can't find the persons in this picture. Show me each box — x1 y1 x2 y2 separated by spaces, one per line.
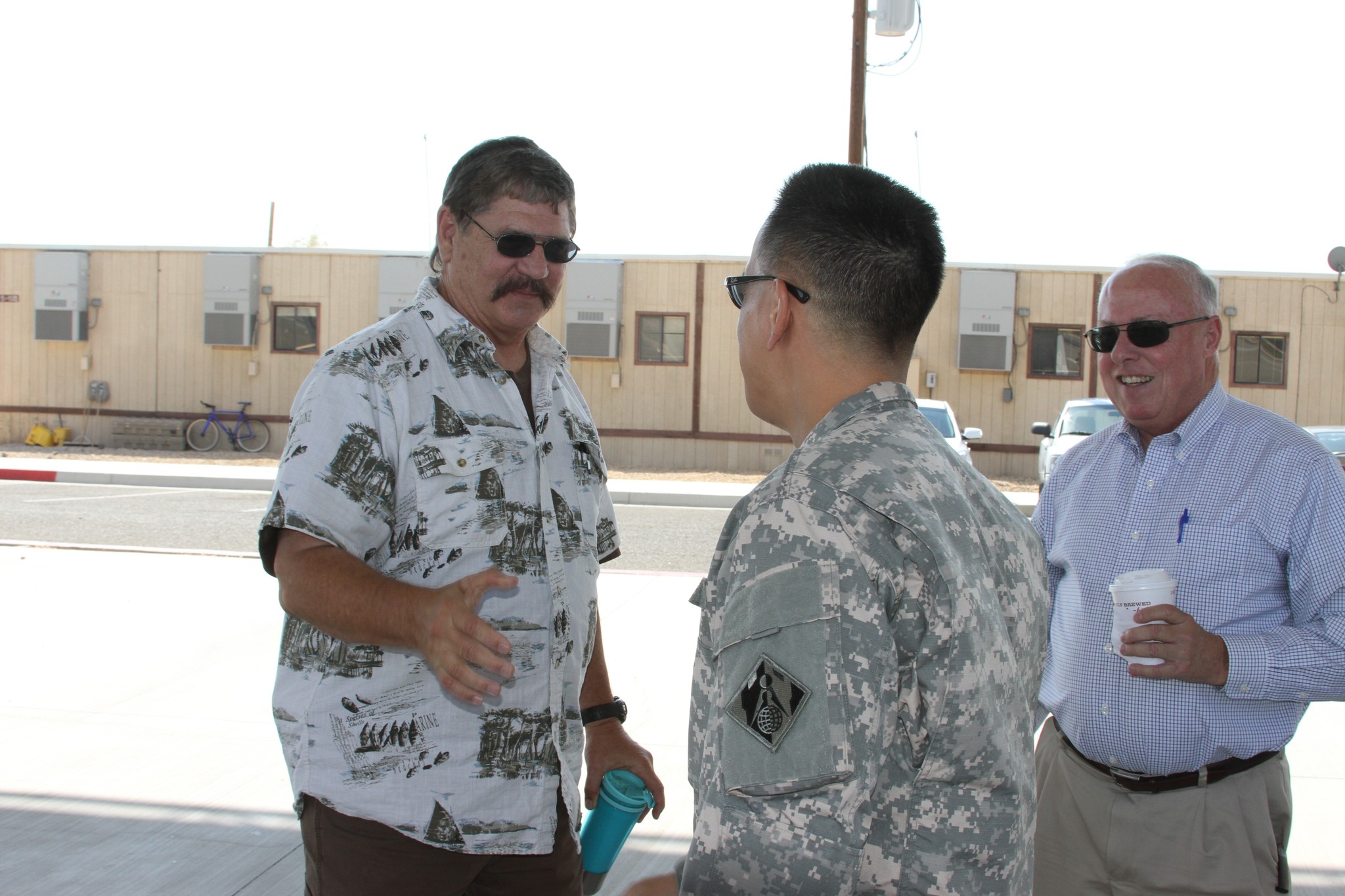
621 164 1052 895
256 136 664 896
1028 254 1345 896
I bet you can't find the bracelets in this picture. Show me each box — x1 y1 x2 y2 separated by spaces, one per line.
581 695 627 725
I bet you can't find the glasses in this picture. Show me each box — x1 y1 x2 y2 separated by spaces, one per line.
726 275 810 309
454 208 580 263
1084 315 1210 353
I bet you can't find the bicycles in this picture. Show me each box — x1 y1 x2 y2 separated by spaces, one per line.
187 400 271 452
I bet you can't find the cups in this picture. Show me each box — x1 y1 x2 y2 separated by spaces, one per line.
577 770 656 896
1108 567 1179 667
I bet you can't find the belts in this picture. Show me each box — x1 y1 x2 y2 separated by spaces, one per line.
1052 715 1282 793
